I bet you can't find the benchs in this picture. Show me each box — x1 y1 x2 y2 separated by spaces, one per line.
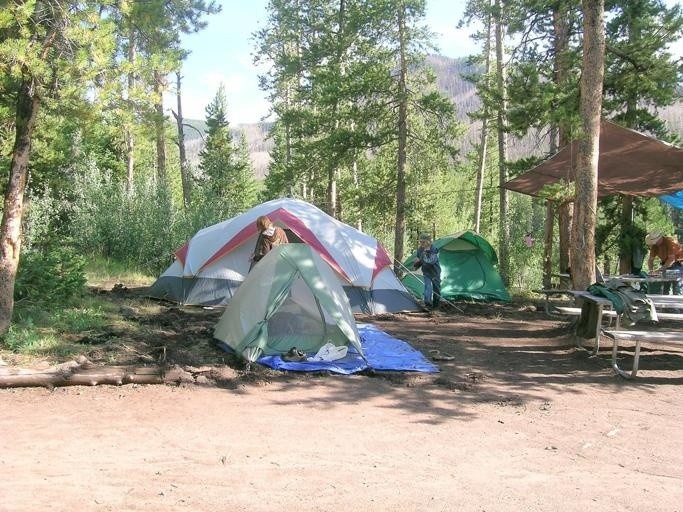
532 268 683 387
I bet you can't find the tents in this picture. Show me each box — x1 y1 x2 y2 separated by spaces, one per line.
399 229 512 303
140 197 422 314
213 242 370 370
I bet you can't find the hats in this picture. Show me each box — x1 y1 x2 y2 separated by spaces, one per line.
417 233 431 242
644 228 662 245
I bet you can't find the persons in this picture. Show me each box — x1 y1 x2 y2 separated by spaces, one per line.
645 231 683 294
256 215 288 255
414 233 442 310
